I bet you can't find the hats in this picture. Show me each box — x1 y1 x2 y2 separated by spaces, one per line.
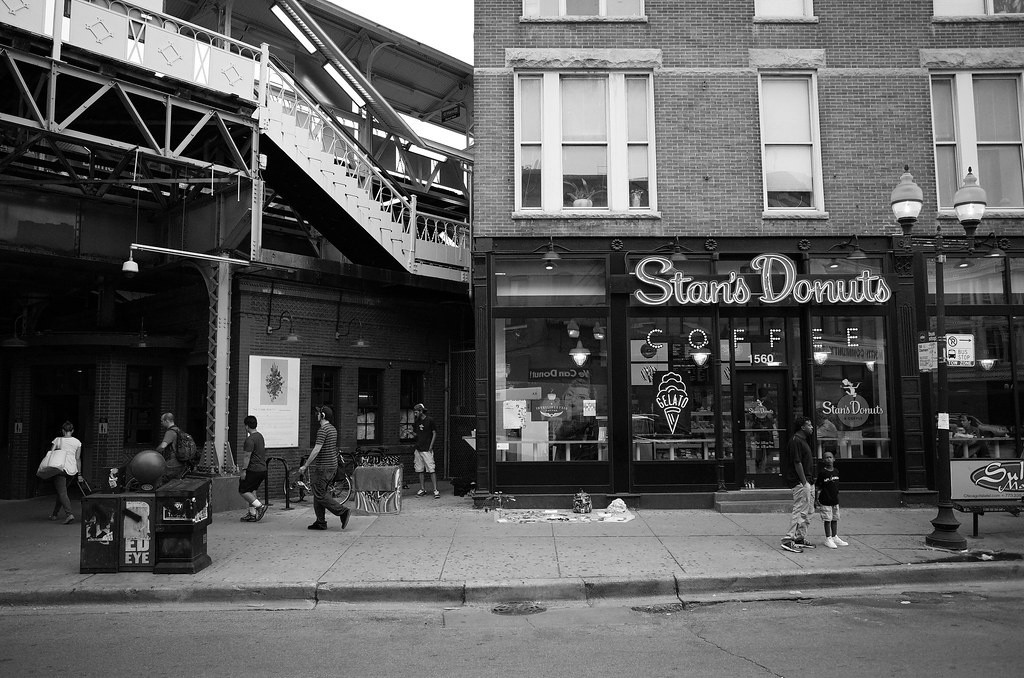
315 405 332 418
413 403 427 413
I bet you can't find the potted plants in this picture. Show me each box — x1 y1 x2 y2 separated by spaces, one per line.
563 178 607 207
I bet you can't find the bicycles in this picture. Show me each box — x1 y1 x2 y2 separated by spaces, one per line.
283 455 352 506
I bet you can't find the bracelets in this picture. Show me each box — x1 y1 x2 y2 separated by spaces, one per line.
78 475 81 476
242 468 247 471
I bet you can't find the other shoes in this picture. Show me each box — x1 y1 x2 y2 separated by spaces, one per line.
256 504 268 521
433 490 440 498
241 513 256 522
340 509 351 529
64 514 75 524
414 490 426 498
48 514 57 521
307 520 327 530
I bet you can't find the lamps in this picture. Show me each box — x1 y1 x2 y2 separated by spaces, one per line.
568 340 591 367
567 318 580 338
976 232 1006 258
542 235 562 262
669 235 687 261
594 322 605 339
840 234 867 259
266 310 298 341
688 348 712 366
335 317 369 348
813 347 832 366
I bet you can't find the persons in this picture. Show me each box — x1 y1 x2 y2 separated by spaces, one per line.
298 405 352 530
816 451 848 548
238 416 269 522
816 412 839 456
955 415 982 458
781 416 816 553
156 413 183 487
48 421 84 523
748 388 776 474
413 403 440 499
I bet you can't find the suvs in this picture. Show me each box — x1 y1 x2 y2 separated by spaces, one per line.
937 413 1009 439
579 413 706 458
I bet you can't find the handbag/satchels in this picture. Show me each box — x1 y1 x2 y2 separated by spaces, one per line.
333 463 345 482
36 437 65 478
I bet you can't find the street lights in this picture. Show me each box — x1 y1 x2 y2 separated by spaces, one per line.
891 164 987 549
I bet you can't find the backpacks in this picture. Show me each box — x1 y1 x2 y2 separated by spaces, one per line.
167 429 197 461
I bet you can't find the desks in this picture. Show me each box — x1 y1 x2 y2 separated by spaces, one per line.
816 437 890 458
950 436 1024 459
548 440 608 462
632 438 716 460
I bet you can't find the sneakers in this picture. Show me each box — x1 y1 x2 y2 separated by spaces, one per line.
781 538 803 553
824 536 838 549
833 534 849 546
795 539 816 548
